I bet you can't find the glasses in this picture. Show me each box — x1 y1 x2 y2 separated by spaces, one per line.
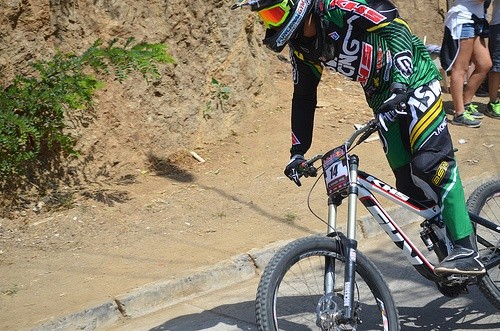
259 0 292 29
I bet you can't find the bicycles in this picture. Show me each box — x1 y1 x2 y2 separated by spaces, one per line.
255 102 500 331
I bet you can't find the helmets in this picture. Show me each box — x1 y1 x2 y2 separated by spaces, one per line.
250 0 335 63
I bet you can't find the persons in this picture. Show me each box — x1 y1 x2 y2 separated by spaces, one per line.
247 0 488 274
439 0 492 128
474 0 500 119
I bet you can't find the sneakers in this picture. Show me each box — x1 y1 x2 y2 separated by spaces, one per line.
464 103 484 118
483 101 500 119
452 110 482 127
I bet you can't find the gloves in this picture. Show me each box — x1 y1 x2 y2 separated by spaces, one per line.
284 153 315 187
377 83 408 131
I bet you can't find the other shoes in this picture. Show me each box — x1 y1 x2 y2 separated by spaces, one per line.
474 84 489 96
433 233 486 277
